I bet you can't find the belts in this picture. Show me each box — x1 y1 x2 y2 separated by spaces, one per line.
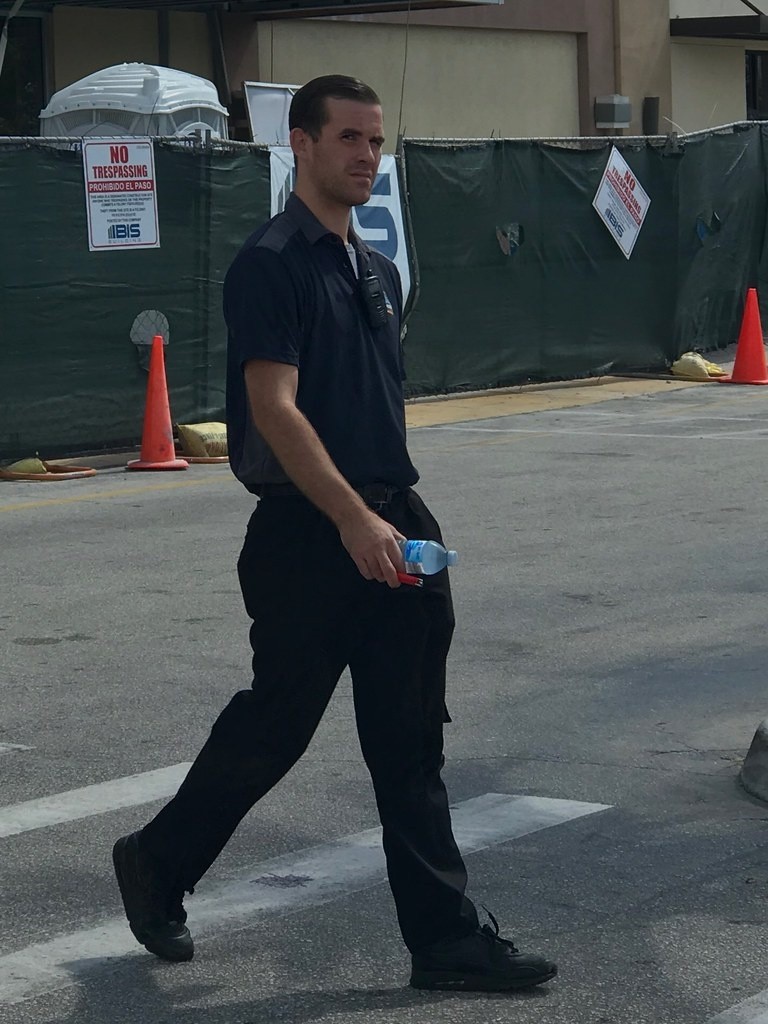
258 480 409 503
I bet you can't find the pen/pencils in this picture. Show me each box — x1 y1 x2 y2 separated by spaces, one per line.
397 572 424 588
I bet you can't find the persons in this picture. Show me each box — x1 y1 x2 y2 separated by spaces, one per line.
114 73 559 995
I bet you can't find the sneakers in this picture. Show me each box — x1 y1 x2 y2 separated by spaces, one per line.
409 905 553 991
113 830 194 961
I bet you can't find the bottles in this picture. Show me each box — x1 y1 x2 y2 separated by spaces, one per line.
395 539 458 575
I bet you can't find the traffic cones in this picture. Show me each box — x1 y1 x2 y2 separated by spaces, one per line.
716 289 768 387
127 335 194 471
